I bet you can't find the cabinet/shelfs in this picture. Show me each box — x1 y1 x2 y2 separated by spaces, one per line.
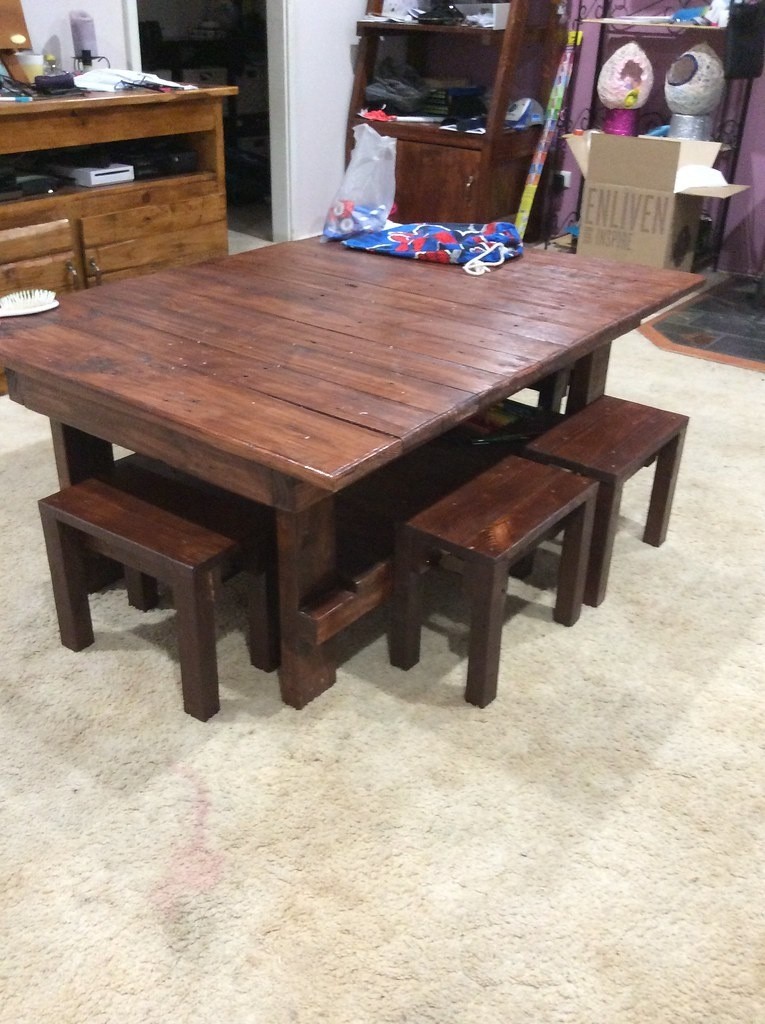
140 40 269 130
0 78 241 393
344 1 568 244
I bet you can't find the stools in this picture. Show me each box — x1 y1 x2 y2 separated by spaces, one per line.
40 454 282 723
386 440 602 712
508 400 692 608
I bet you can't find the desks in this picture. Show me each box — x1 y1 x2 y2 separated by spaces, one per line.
0 221 706 709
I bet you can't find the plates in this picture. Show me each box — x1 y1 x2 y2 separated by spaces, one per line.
0 300 60 318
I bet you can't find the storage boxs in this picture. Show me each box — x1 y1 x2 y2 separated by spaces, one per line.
563 129 752 269
